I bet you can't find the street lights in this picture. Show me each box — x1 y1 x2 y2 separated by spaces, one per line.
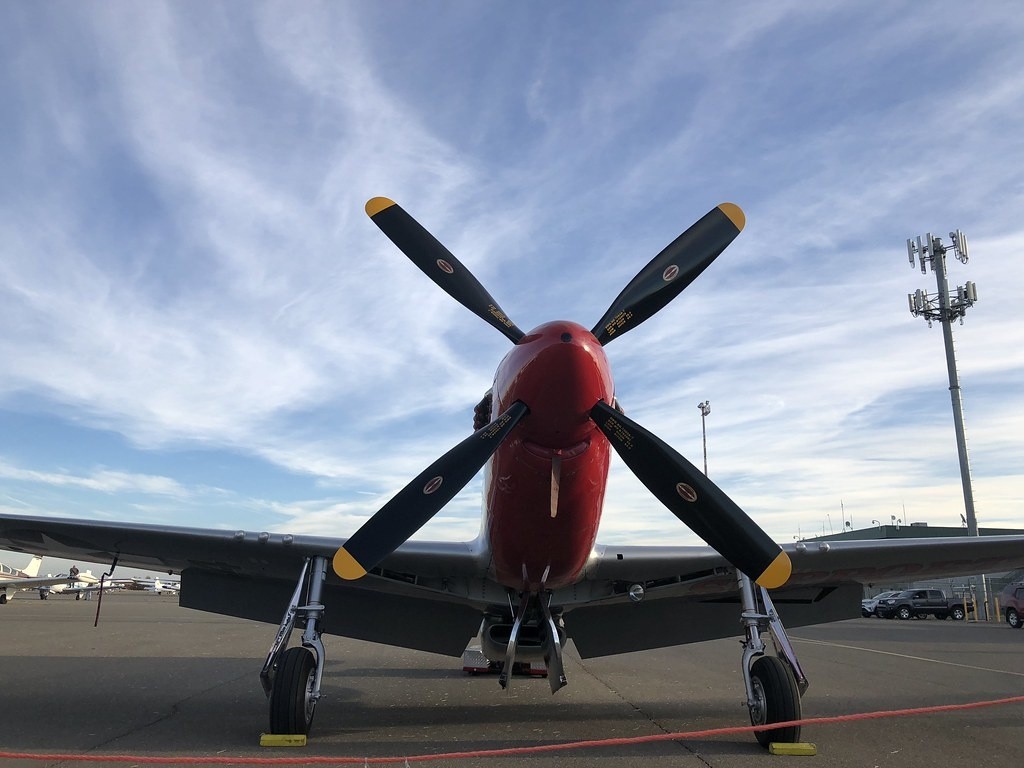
696 399 712 479
906 229 989 623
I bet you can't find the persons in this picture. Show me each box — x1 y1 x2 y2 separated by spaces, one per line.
68 565 79 588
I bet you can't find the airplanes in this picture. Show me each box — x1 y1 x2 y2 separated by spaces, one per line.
1 551 180 607
0 194 1024 768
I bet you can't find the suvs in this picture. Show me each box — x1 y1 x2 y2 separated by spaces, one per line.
862 591 905 618
999 582 1024 629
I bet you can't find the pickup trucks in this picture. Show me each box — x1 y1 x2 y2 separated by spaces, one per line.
876 588 975 621
